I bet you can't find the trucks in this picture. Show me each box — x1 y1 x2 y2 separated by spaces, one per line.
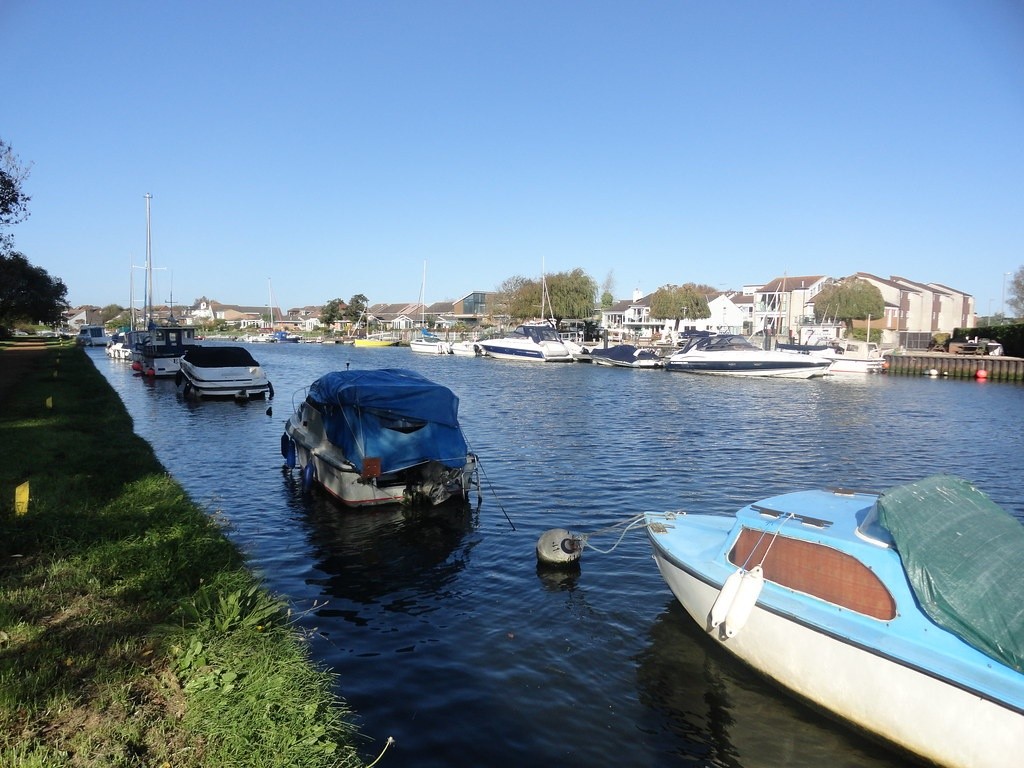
958 342 1004 356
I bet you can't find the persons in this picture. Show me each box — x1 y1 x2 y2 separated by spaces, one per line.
149 319 156 345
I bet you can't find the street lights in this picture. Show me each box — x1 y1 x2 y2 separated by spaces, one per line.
1002 271 1013 326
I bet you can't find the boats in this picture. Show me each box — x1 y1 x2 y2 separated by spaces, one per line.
315 337 324 343
342 340 354 344
178 347 275 401
126 291 202 376
450 339 489 356
76 325 112 346
322 340 335 344
561 339 583 360
280 368 482 509
641 478 1024 768
274 331 301 343
774 338 836 357
590 344 663 368
235 334 266 342
822 338 886 373
306 341 312 343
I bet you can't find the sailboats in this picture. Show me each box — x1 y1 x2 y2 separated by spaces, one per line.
663 271 832 380
105 193 154 361
408 260 453 354
259 278 277 343
355 301 399 347
478 256 574 363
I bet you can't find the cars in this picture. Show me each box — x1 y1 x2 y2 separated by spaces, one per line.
756 329 774 337
601 330 623 342
219 327 227 331
677 332 717 348
